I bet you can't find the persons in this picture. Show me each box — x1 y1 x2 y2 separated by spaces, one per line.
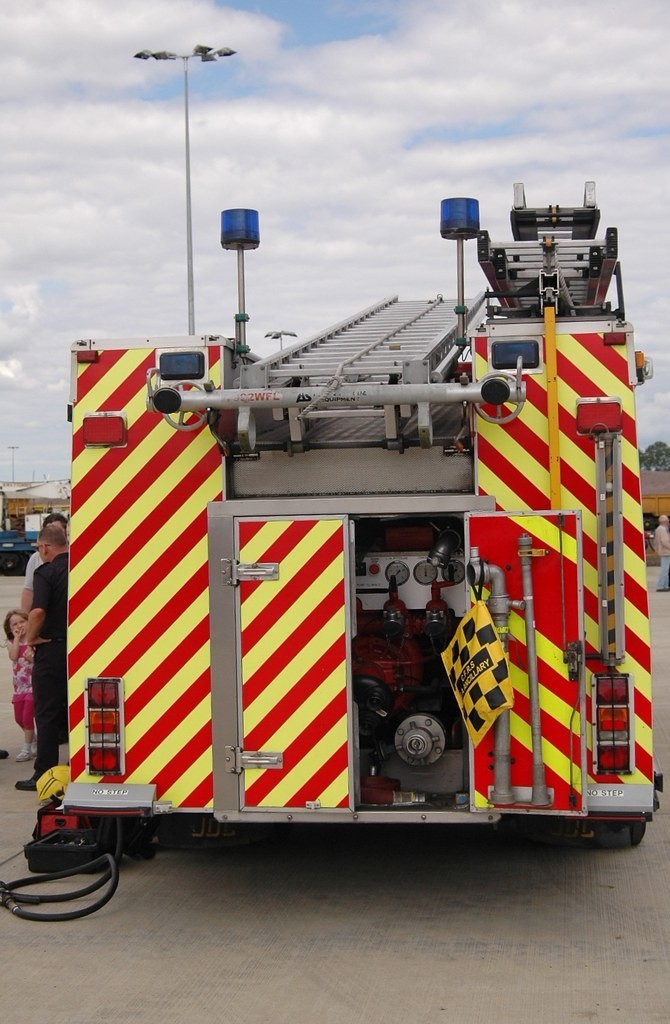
650 514 670 592
13 521 68 791
20 512 70 614
1 608 44 764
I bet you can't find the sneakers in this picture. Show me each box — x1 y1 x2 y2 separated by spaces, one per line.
31 740 38 755
16 751 33 762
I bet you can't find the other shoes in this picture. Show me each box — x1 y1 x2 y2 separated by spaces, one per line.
0 749 9 760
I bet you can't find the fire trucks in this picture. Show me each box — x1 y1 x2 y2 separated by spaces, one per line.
25 174 662 875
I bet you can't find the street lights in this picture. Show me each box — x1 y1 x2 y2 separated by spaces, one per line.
136 44 234 336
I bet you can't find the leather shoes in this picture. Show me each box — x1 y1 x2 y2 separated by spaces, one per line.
15 779 37 790
